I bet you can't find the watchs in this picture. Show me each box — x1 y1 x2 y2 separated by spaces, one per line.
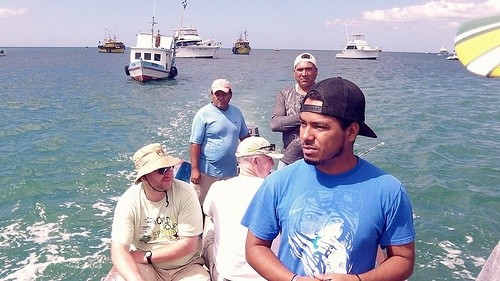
145 251 154 264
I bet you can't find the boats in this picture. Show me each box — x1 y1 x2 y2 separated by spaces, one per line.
0 49 7 57
173 14 221 59
332 30 383 60
232 29 252 55
97 27 126 54
124 0 178 84
436 46 460 61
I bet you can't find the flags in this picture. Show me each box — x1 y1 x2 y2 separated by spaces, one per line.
182 0 187 9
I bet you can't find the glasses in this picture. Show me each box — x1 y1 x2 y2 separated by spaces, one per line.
260 144 275 151
155 165 175 175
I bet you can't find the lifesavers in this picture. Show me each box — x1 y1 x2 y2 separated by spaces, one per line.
155 36 160 47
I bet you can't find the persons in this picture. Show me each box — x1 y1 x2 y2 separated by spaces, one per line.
111 142 213 281
240 78 416 281
269 53 318 169
191 78 251 241
203 136 287 281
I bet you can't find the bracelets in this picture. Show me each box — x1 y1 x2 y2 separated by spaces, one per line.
355 274 361 281
291 274 297 281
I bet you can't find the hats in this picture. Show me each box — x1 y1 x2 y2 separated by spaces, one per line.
235 135 284 159
294 52 318 69
300 76 378 138
211 78 232 94
134 142 182 185
